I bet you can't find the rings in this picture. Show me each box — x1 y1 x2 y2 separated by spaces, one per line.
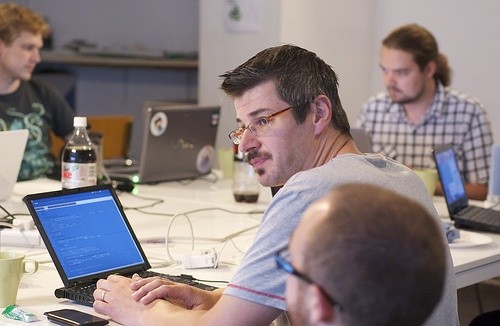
102 291 108 302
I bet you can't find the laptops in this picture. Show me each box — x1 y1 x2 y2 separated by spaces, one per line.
101 104 222 183
432 143 500 234
23 183 219 307
0 129 30 202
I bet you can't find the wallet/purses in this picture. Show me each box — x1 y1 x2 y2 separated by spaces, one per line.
42 309 109 326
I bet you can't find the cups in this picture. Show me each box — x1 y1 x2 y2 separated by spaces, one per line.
65 132 103 182
411 167 439 200
0 251 39 308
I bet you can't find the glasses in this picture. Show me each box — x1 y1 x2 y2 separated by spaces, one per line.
274 246 343 313
227 90 326 145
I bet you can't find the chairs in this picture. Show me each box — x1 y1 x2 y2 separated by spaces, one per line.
50 116 133 162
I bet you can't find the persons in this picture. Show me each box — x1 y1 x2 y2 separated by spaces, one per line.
353 23 494 201
93 45 459 326
0 1 98 183
275 183 447 326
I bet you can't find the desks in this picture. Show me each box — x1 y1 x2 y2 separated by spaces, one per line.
0 168 500 326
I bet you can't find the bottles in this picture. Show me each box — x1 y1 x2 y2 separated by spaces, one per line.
61 116 98 190
232 132 261 203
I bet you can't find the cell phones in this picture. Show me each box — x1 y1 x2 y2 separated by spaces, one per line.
43 308 109 326
